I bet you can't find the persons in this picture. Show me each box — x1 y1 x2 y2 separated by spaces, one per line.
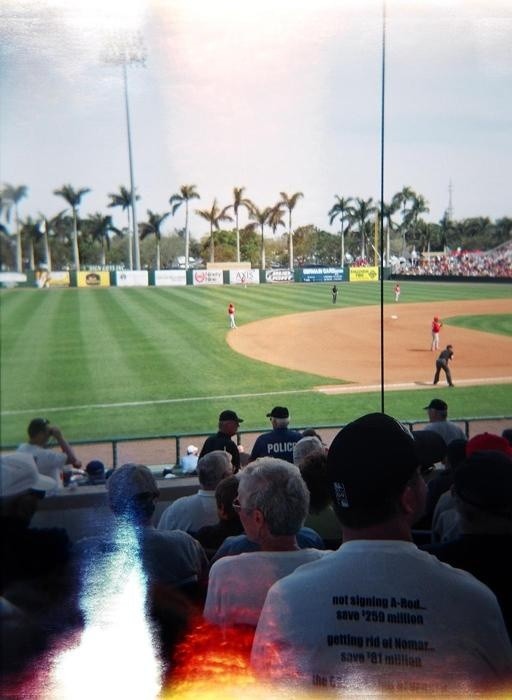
431 316 443 351
228 303 238 329
2 398 512 696
332 285 338 304
433 345 454 387
395 284 400 302
350 243 512 277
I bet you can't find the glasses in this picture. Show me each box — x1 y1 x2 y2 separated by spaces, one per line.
232 498 254 512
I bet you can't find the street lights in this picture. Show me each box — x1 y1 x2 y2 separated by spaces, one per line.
100 25 151 270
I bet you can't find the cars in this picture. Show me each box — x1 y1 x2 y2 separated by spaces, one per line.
177 262 186 269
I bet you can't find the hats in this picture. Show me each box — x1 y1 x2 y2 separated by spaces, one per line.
103 461 161 508
267 407 289 419
219 411 243 423
464 431 512 456
325 411 427 511
424 399 448 411
28 418 50 437
85 460 104 475
187 444 198 453
455 449 512 512
